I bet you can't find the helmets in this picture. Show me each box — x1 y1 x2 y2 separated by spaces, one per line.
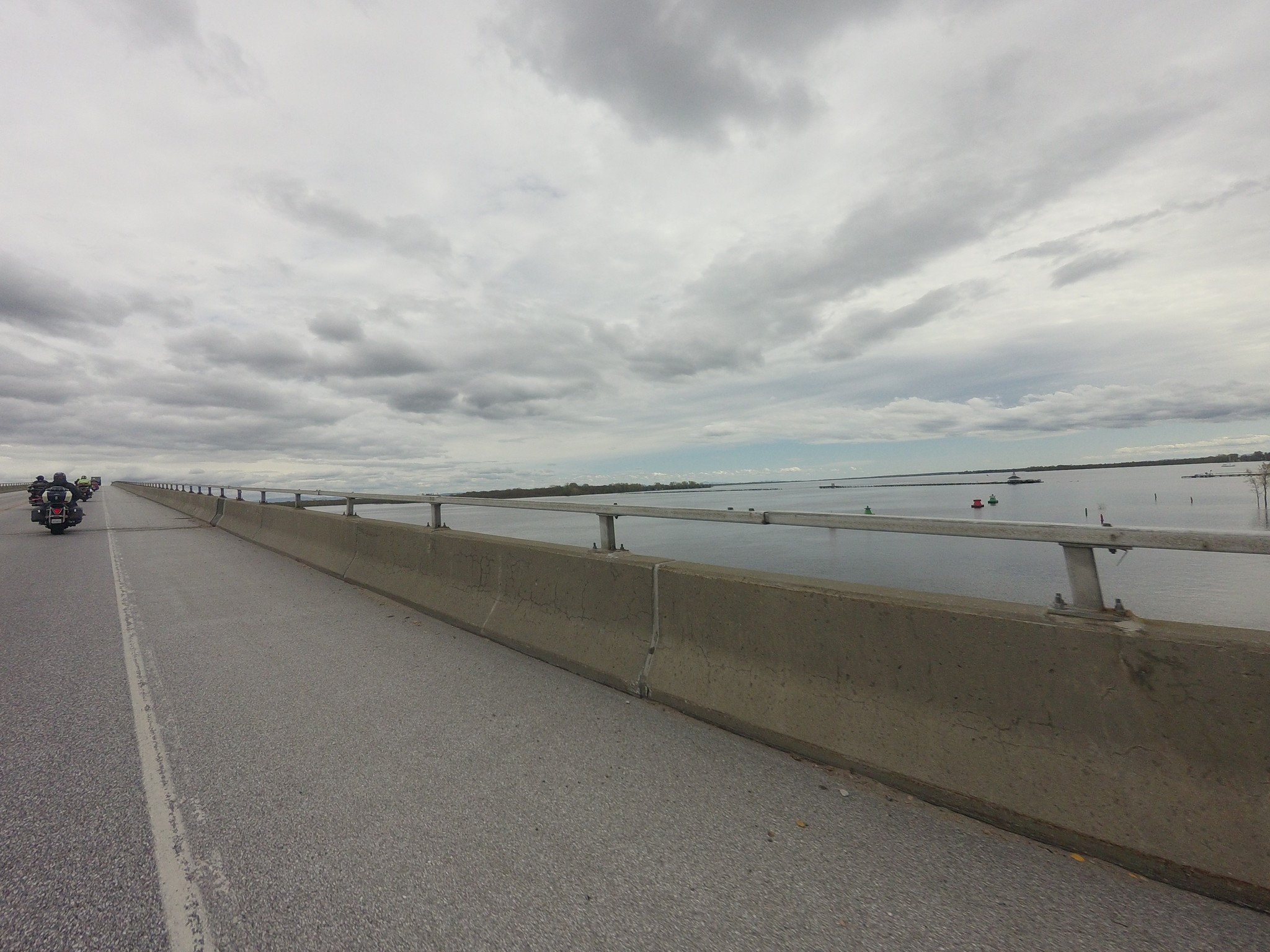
82 476 86 478
53 472 66 480
37 476 44 480
92 478 95 480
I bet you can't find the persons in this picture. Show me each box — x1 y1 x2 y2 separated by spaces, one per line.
1101 518 1104 524
90 478 99 489
77 476 93 498
28 475 50 500
1154 493 1157 500
1101 514 1103 519
39 471 81 525
1190 497 1193 504
74 479 80 486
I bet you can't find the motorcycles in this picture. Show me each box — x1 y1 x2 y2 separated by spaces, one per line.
90 480 99 492
31 486 83 535
77 482 93 502
27 487 48 507
